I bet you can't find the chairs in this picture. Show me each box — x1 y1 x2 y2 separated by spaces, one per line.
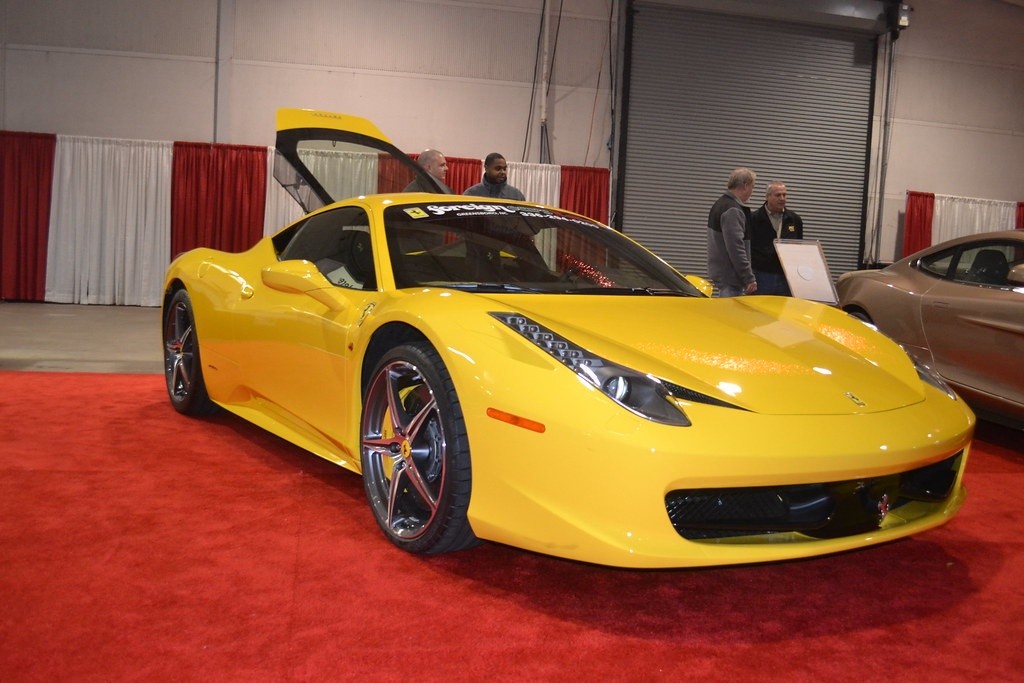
338 229 373 267
966 249 1012 285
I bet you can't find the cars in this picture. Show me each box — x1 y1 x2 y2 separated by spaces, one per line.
838 227 1023 432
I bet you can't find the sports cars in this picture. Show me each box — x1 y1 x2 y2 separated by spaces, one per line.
161 105 974 570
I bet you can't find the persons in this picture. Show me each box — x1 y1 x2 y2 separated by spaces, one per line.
707 168 757 298
751 182 803 297
402 148 452 251
462 153 527 258
